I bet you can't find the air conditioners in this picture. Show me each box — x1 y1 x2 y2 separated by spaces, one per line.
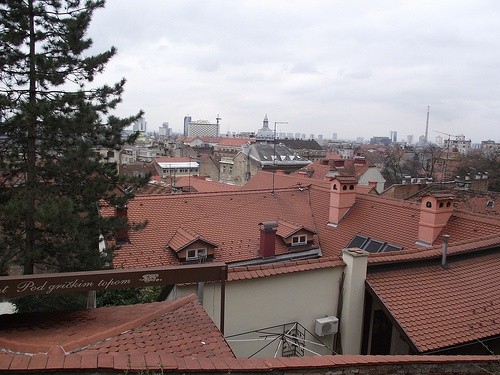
315 316 339 337
